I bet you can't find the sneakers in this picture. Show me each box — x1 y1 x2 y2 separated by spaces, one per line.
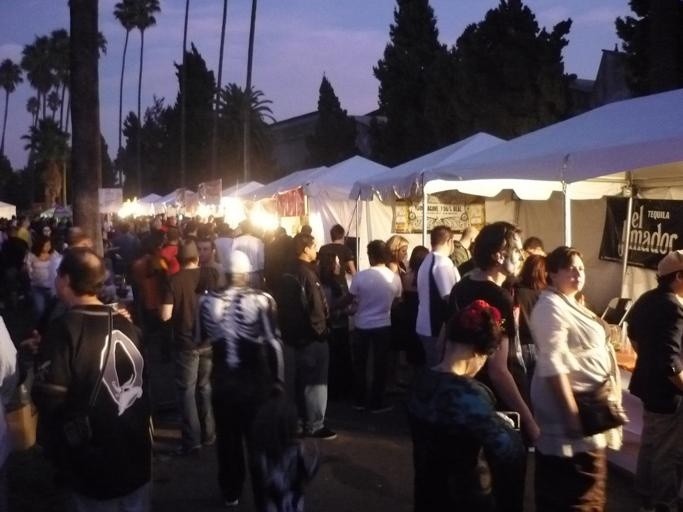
370 404 393 414
219 477 239 505
350 404 369 410
304 428 337 439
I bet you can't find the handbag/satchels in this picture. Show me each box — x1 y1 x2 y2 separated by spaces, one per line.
573 374 630 439
176 241 199 259
223 250 252 273
41 409 93 480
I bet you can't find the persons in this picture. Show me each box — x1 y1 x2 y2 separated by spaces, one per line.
232 218 319 286
436 220 541 512
522 237 550 258
414 225 461 371
315 224 358 293
529 246 630 512
280 233 336 441
448 225 479 268
158 238 216 456
30 246 151 512
0 214 73 248
101 210 230 272
384 236 407 293
502 254 546 453
625 250 682 512
196 250 284 512
24 236 59 292
402 245 429 291
404 300 528 512
0 237 35 342
56 235 136 326
0 314 38 510
319 250 356 404
347 238 404 414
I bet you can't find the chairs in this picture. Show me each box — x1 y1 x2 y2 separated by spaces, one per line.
600 298 634 333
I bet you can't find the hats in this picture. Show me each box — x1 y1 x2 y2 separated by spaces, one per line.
656 250 683 278
